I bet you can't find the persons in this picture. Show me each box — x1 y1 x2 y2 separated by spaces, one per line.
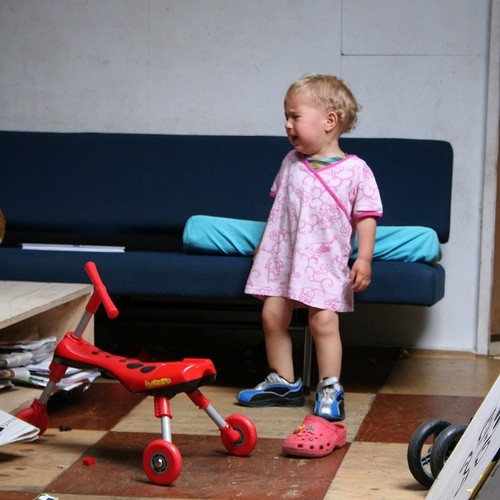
236 73 384 420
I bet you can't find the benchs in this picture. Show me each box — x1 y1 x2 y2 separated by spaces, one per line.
0 129 453 393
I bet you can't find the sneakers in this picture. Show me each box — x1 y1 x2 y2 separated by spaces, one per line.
236 368 305 407
313 376 345 421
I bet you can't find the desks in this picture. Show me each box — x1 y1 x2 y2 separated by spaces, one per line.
0 281 96 435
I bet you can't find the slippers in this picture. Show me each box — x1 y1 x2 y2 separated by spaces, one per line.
282 415 346 457
